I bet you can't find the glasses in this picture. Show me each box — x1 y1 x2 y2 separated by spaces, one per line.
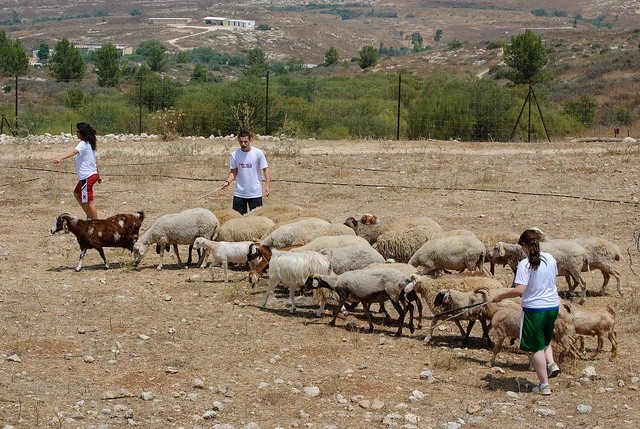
76 130 81 132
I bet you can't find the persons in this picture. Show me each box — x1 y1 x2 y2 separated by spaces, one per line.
492 229 560 396
220 131 271 215
52 122 102 221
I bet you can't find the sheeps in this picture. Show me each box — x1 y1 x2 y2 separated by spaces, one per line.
293 224 355 243
434 230 478 241
371 229 436 259
192 237 253 282
361 213 377 225
291 235 370 252
566 304 617 362
261 217 330 244
242 204 305 216
404 275 505 344
366 263 423 334
489 241 591 304
476 230 519 288
485 305 577 368
434 289 493 347
330 244 385 271
213 216 275 240
298 268 409 336
408 237 489 273
527 227 626 297
50 212 145 272
215 208 240 225
132 208 219 270
247 243 329 315
467 286 522 322
341 216 441 245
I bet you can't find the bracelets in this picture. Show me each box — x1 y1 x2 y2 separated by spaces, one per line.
225 180 230 186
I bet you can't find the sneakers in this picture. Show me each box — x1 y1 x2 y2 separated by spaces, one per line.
531 384 550 395
547 362 560 378
